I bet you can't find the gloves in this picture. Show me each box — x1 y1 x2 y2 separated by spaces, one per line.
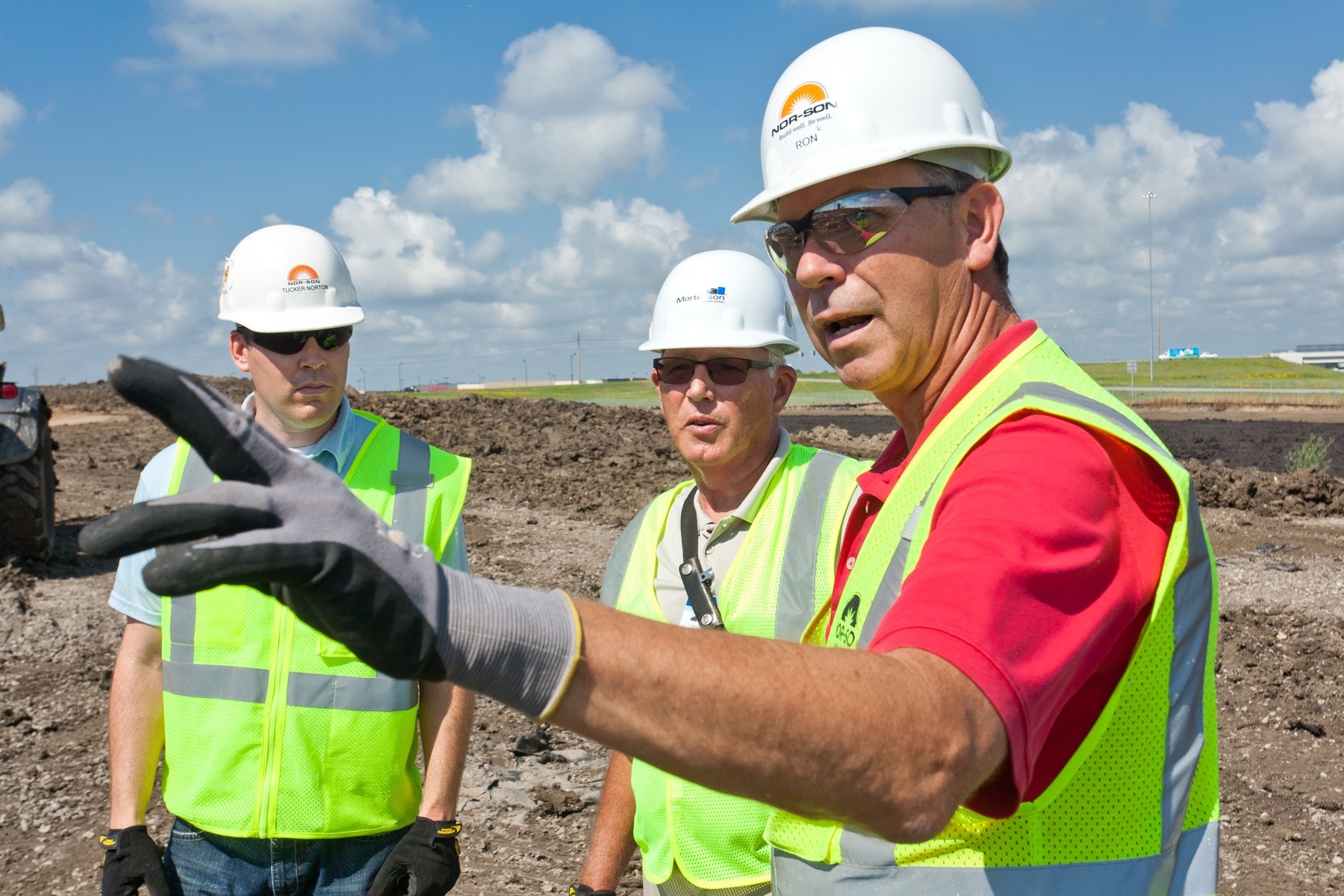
366 817 465 896
98 824 193 896
68 354 593 731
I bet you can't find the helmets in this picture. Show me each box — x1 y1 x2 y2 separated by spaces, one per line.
727 25 1016 220
638 248 813 357
216 223 367 332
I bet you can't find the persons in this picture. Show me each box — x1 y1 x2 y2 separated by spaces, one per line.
80 26 1219 896
99 224 472 896
567 250 882 896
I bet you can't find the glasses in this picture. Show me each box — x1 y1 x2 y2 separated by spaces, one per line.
647 355 779 388
760 181 962 277
236 327 356 355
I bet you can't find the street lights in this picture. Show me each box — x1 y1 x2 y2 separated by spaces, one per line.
548 372 557 386
570 353 576 386
398 362 403 392
360 368 365 391
522 359 527 388
1142 191 1158 381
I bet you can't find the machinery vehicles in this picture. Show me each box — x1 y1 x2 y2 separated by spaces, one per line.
0 304 65 573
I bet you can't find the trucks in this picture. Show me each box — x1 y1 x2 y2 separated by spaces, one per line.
1158 347 1200 361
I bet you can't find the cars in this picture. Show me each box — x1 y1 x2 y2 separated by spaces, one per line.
404 386 419 393
1200 352 1218 360
